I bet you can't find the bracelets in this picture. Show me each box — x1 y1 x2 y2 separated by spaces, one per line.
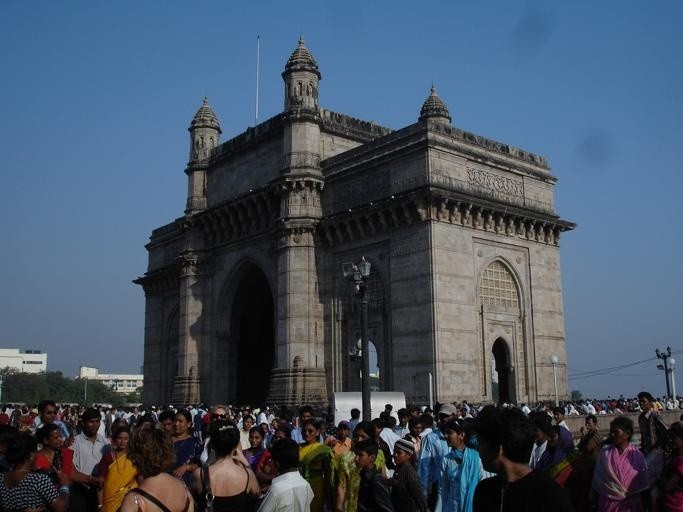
58 485 71 495
96 503 103 509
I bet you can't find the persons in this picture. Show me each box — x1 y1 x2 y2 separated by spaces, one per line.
243 427 266 468
553 407 568 429
590 417 650 512
256 438 315 512
118 405 159 422
393 409 410 438
411 406 433 415
404 419 422 453
519 402 530 415
0 403 38 430
326 421 352 457
531 401 563 412
120 422 194 512
653 395 668 412
105 407 118 435
482 401 516 408
417 404 458 503
291 406 323 443
372 411 387 428
536 425 583 504
668 396 683 410
473 406 574 512
256 425 291 507
577 414 602 474
527 411 552 468
455 400 482 419
354 440 394 512
418 415 435 439
335 421 387 512
98 404 111 419
347 409 361 438
371 419 390 467
189 419 259 512
391 434 430 512
240 405 256 415
297 419 335 511
68 408 111 512
435 419 485 512
35 400 70 442
638 392 670 488
384 404 396 425
0 431 71 512
56 404 68 421
96 425 139 512
71 405 84 435
563 394 640 415
168 409 202 488
159 411 175 432
257 404 279 432
199 405 230 466
33 422 64 492
379 416 400 465
655 421 683 512
229 405 241 427
240 415 255 450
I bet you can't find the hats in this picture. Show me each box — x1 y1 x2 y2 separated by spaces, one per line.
439 404 457 415
395 439 414 453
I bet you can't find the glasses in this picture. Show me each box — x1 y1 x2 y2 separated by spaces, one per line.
42 410 57 414
212 413 227 418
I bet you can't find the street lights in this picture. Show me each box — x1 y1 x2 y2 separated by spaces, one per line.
655 346 672 398
549 354 559 406
342 255 372 421
669 358 676 400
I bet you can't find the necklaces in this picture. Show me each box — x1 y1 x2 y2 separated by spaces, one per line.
114 450 128 474
